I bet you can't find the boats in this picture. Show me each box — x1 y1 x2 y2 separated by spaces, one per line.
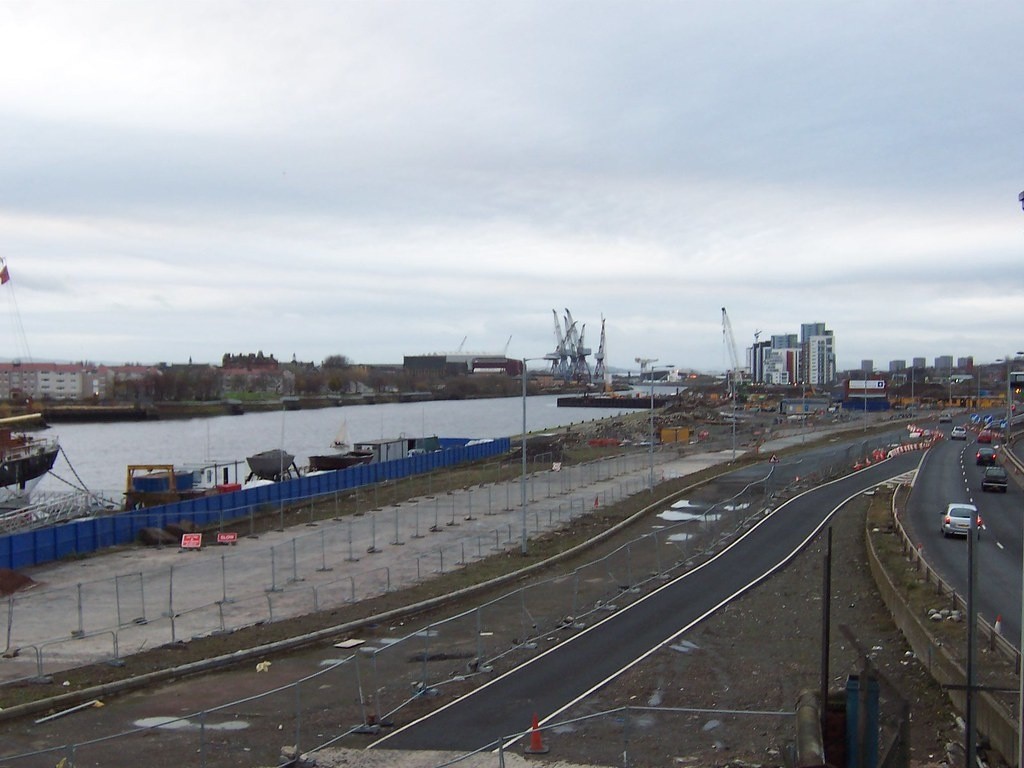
123 483 242 511
307 449 376 472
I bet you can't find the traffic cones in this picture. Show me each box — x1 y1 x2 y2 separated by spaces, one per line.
523 711 550 754
993 613 1002 635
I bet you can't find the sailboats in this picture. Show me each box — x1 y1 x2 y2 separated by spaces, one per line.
1 254 63 490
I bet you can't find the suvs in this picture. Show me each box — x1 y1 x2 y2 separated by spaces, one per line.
981 466 1009 493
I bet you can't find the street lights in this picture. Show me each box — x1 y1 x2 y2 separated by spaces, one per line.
911 364 923 419
520 356 559 558
862 369 880 433
949 377 959 408
730 370 745 465
649 365 675 492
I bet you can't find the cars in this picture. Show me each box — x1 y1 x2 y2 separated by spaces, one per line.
951 425 968 440
939 502 982 540
938 411 953 423
975 447 997 466
977 431 992 444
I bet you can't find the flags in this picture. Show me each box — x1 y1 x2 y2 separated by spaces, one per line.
0 265 10 284
